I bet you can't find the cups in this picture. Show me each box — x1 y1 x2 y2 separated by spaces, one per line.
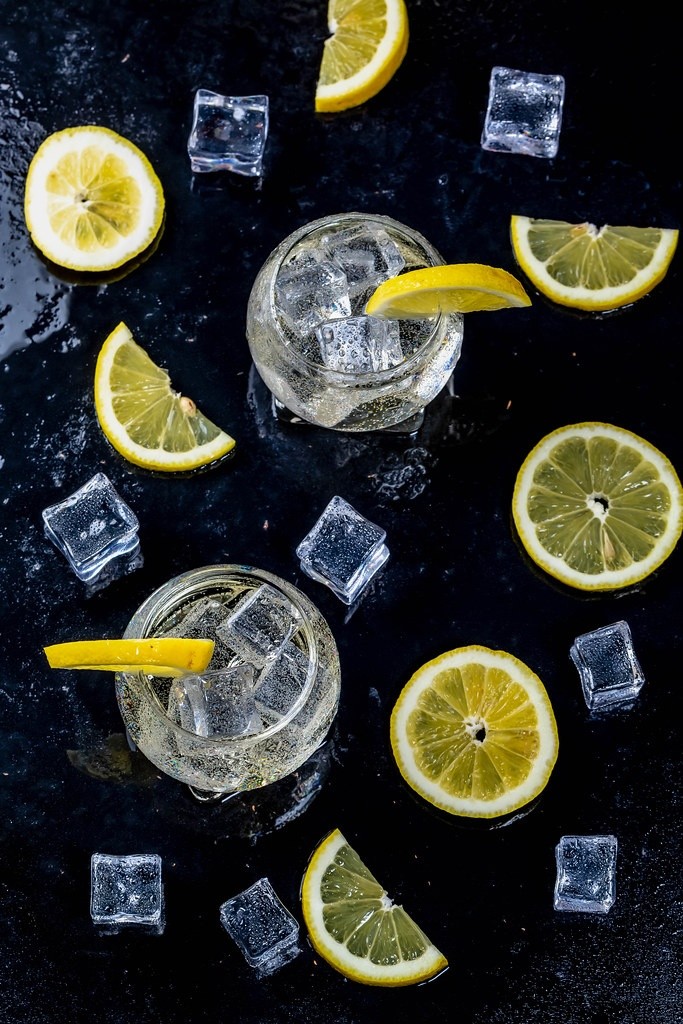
116 564 338 792
247 212 463 434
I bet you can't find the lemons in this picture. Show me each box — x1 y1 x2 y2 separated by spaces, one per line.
25 0 683 984
